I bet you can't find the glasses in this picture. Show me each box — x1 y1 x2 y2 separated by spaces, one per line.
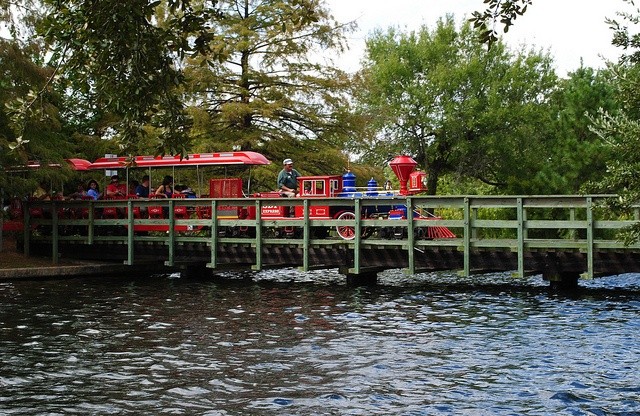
113 180 118 182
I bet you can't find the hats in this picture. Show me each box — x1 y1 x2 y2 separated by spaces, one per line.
283 158 294 165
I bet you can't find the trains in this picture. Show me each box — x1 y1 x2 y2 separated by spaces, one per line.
1 152 457 239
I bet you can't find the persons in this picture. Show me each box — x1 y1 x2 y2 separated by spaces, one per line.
136 174 152 199
33 182 50 200
87 180 101 200
68 183 86 199
106 176 128 197
278 159 302 193
154 176 181 199
